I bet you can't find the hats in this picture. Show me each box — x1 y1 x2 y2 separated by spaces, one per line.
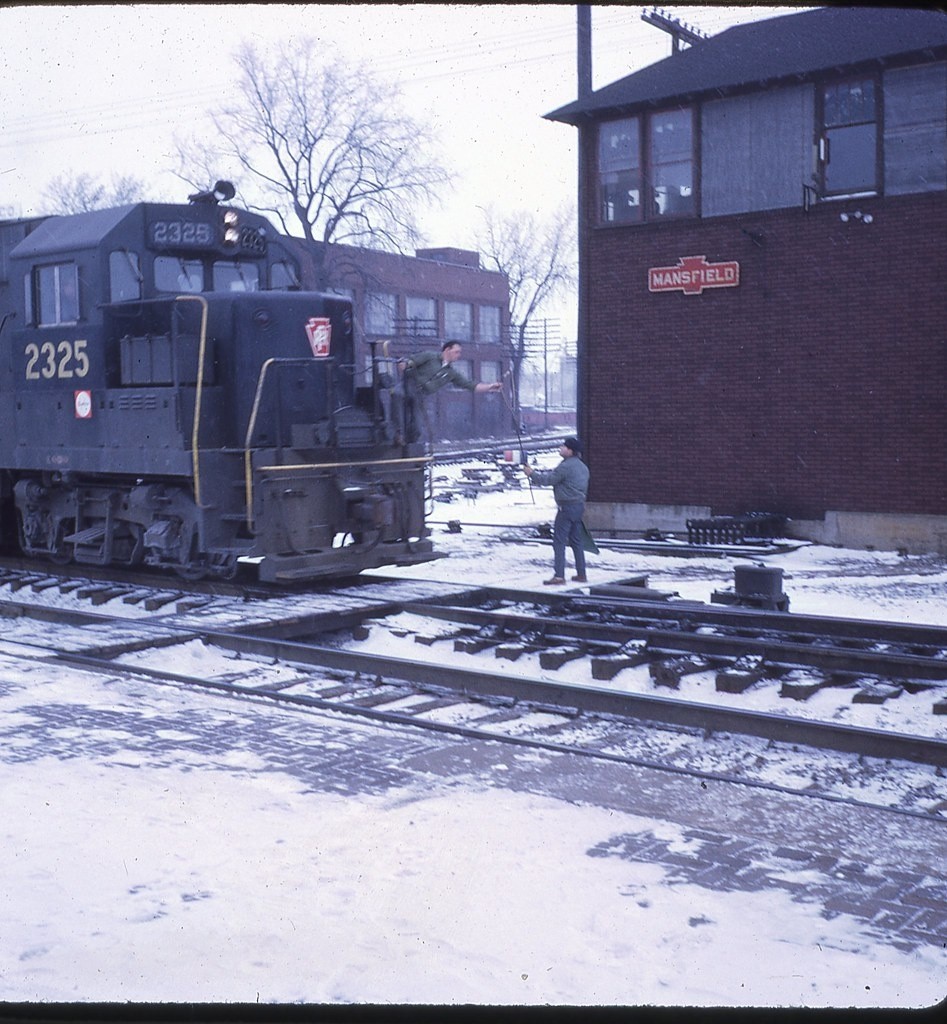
565 438 580 453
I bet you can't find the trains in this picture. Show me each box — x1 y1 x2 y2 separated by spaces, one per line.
0 178 451 584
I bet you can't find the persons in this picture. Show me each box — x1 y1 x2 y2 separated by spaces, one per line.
392 339 501 444
525 437 590 585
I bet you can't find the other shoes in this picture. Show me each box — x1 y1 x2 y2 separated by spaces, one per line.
543 578 565 585
572 576 585 582
